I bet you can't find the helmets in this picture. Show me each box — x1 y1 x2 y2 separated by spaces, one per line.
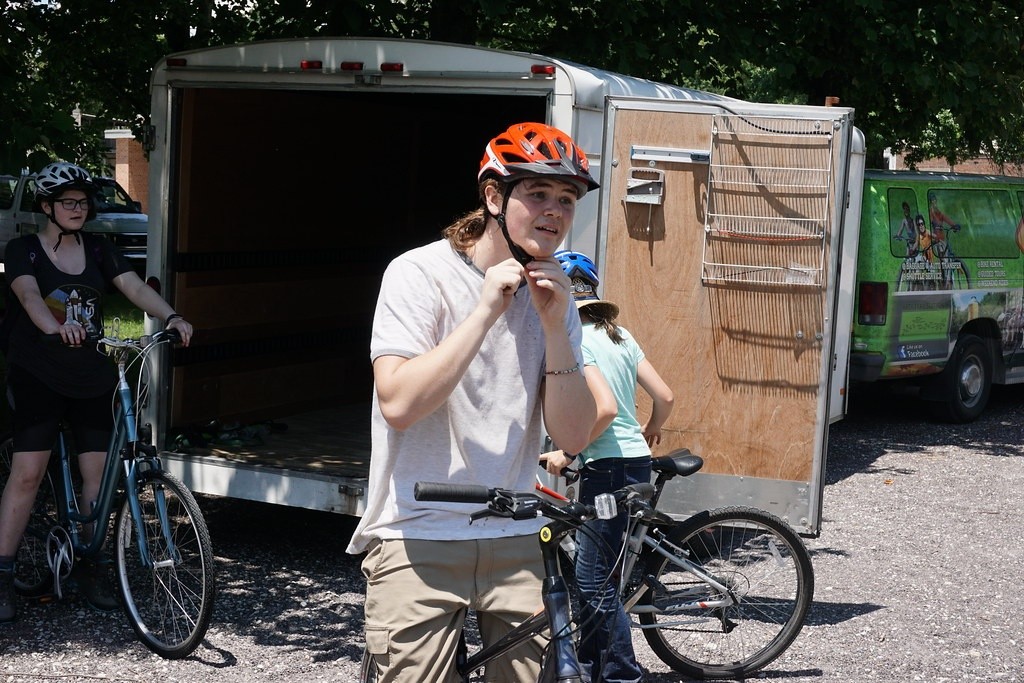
35 159 95 199
478 122 604 202
553 250 601 287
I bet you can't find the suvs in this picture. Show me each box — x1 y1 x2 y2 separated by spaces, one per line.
1 173 147 301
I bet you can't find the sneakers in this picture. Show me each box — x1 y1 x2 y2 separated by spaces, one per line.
1 567 18 621
70 562 120 613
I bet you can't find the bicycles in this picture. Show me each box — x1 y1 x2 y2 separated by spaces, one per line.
531 448 817 682
10 326 216 661
361 483 675 683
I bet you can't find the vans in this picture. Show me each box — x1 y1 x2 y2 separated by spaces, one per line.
839 165 1024 425
137 38 868 551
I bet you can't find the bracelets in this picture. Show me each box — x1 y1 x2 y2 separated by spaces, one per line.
165 314 184 325
563 452 577 461
543 360 580 374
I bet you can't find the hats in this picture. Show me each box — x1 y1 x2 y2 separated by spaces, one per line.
572 283 619 320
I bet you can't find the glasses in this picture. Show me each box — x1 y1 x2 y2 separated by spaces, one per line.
47 196 95 212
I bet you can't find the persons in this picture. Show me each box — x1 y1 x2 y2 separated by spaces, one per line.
895 192 959 290
0 161 193 615
354 120 605 683
538 249 674 683
